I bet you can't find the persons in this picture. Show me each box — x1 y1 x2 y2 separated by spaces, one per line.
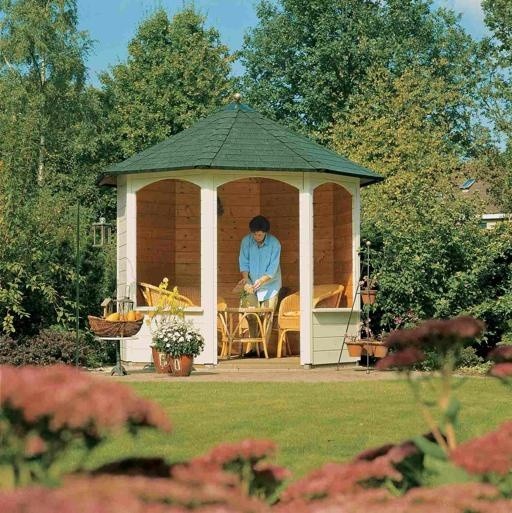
237 214 286 359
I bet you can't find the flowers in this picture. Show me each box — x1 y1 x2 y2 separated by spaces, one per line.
359 274 379 289
143 277 205 359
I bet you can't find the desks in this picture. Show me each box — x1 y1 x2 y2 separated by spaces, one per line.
95 336 138 375
223 305 275 359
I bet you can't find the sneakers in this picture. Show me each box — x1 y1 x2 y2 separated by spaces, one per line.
243 349 265 357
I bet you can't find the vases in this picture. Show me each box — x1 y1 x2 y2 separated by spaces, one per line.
360 289 377 305
346 340 389 357
344 310 413 341
151 344 193 376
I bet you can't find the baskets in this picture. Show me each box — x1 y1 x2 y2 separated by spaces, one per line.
88 315 144 337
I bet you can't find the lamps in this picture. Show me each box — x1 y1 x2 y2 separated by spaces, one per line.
63 191 112 375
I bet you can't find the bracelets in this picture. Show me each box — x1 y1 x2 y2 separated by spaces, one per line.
260 277 266 283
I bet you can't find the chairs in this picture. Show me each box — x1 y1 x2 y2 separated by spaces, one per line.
276 282 344 358
137 278 227 359
